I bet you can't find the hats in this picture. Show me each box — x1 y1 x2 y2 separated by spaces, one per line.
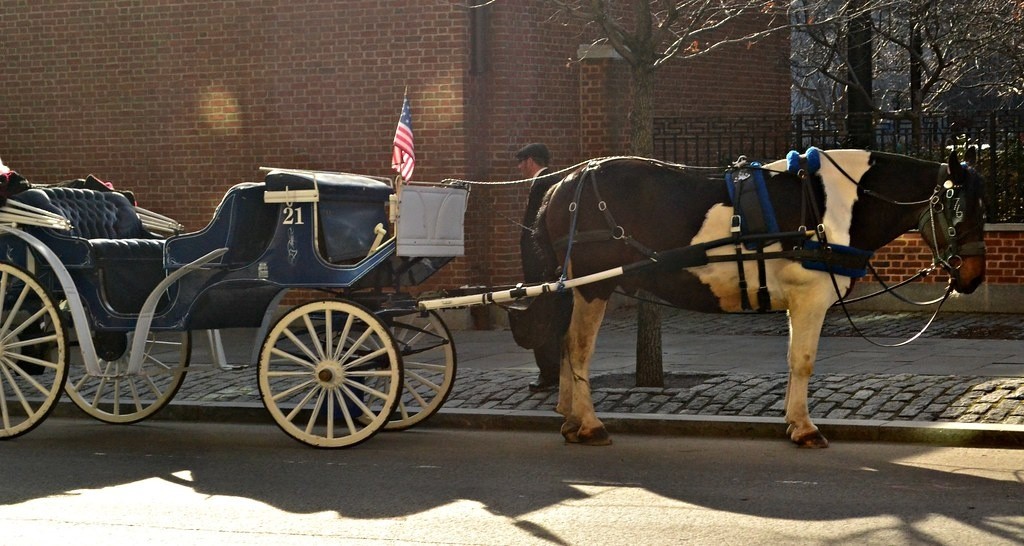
514 143 549 160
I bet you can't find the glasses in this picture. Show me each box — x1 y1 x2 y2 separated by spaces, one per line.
518 157 529 163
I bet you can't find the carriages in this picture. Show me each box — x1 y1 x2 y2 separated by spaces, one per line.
0 146 994 450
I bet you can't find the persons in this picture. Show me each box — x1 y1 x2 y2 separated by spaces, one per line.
514 143 574 393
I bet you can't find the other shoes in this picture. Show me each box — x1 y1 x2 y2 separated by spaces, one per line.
529 375 561 392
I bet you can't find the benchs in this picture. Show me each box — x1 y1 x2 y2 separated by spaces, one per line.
161 181 265 311
11 187 168 315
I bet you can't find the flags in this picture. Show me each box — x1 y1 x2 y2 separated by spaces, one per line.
390 95 414 182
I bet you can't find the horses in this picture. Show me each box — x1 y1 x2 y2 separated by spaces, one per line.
532 148 988 448
